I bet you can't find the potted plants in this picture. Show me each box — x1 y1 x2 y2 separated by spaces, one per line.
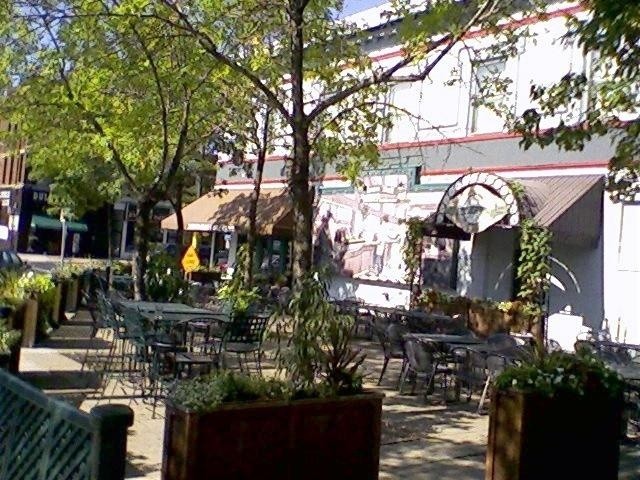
483 348 625 479
159 270 384 480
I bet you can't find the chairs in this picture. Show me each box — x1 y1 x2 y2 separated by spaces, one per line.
329 300 533 401
82 268 270 419
